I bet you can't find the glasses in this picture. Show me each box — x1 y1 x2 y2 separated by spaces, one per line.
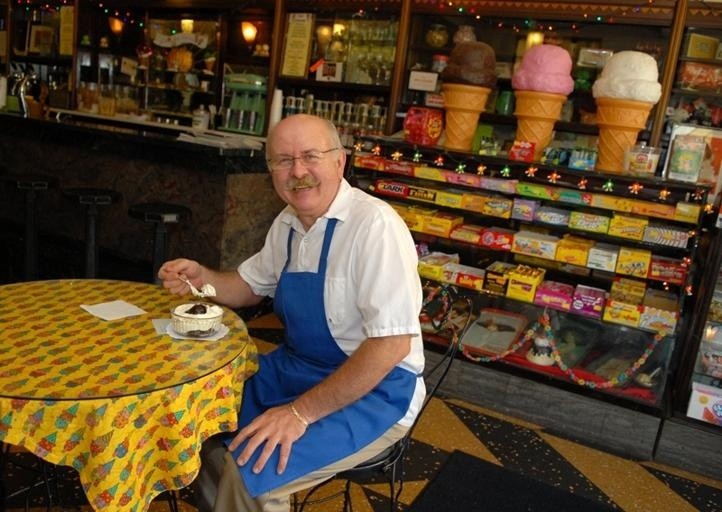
267 148 340 171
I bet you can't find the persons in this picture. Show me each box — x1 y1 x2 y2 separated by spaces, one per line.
157 113 428 512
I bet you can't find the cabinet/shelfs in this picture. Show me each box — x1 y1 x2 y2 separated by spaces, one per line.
347 0 722 432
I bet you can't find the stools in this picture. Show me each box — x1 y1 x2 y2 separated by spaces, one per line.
6 171 193 284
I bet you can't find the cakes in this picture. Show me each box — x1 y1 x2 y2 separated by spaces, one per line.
171 282 223 335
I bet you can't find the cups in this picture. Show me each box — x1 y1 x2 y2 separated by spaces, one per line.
283 93 385 151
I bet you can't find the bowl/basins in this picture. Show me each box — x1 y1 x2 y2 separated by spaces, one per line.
168 304 224 337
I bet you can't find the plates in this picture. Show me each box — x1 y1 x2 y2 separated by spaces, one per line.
164 322 230 341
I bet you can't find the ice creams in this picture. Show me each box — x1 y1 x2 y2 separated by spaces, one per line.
512 44 574 159
440 40 497 150
592 50 661 174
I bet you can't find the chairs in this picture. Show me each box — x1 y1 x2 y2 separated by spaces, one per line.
293 273 472 512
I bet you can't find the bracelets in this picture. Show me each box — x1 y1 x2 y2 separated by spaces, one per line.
288 400 311 427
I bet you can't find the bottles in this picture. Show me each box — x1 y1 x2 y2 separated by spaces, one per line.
627 140 662 178
78 80 141 116
190 104 210 130
425 23 478 49
9 72 49 118
